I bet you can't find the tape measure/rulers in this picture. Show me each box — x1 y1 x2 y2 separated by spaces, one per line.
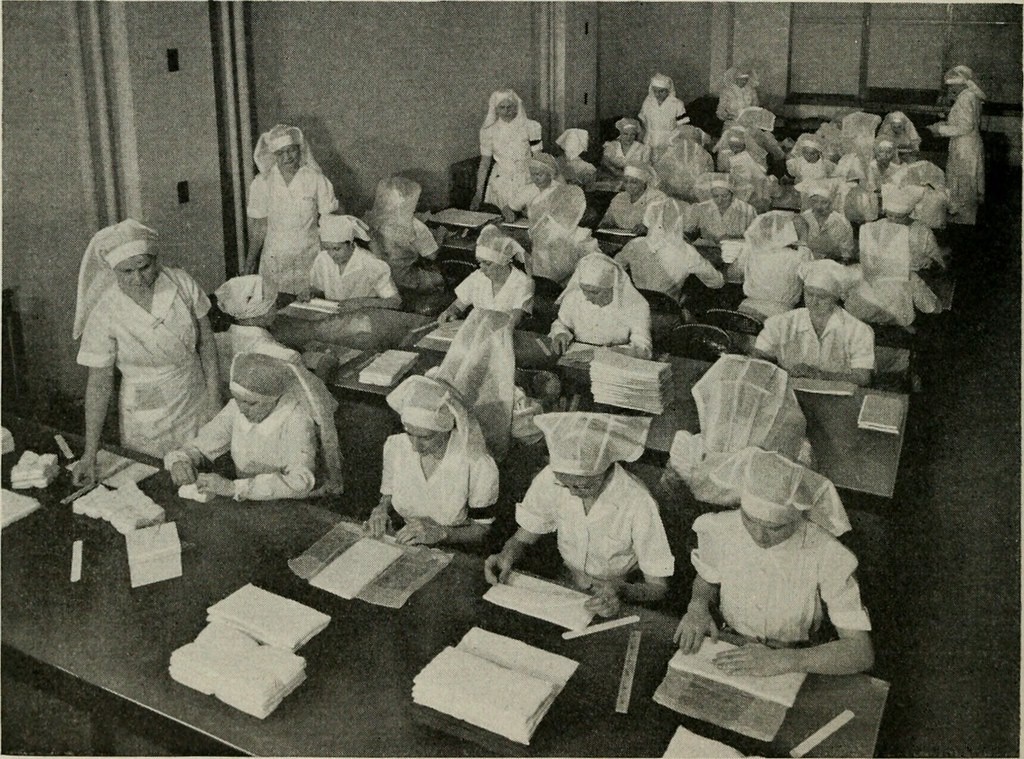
617 631 641 714
58 458 138 506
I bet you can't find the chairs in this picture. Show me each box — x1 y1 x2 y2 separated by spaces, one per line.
324 204 767 604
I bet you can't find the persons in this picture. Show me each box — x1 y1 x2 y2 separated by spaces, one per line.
526 179 602 288
71 217 221 489
210 270 300 401
481 410 676 618
162 335 345 502
927 63 987 225
662 355 825 512
422 306 549 462
366 375 501 550
543 251 654 361
242 124 339 295
362 176 446 288
610 195 726 305
294 214 404 312
738 108 954 325
432 222 536 327
751 257 879 389
463 65 787 239
674 444 876 677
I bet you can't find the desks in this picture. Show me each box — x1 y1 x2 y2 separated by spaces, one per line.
264 288 908 511
418 215 956 317
0 414 891 759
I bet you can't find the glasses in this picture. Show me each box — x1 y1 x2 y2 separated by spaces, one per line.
552 475 605 489
